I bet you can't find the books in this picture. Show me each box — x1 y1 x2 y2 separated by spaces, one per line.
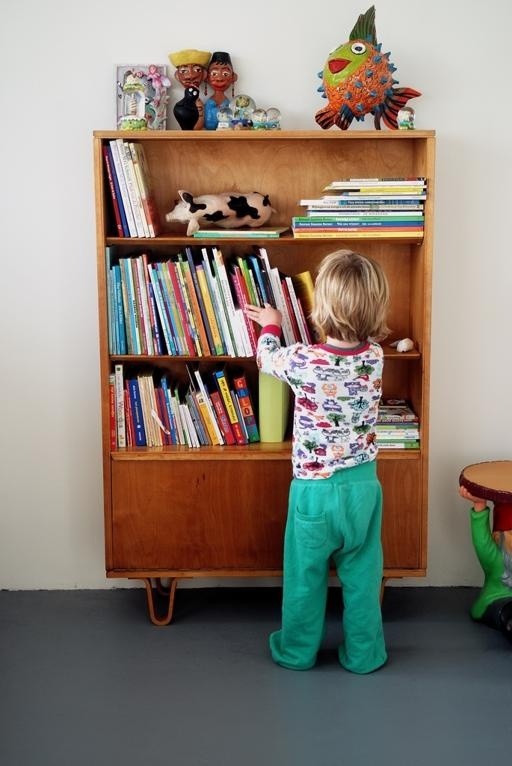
259 369 290 442
105 247 327 357
291 178 427 239
194 227 289 238
109 362 260 449
374 398 420 449
105 139 162 239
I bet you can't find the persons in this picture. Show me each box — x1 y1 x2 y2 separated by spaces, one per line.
242 247 392 676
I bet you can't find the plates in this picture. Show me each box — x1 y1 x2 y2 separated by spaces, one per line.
93 130 435 626
